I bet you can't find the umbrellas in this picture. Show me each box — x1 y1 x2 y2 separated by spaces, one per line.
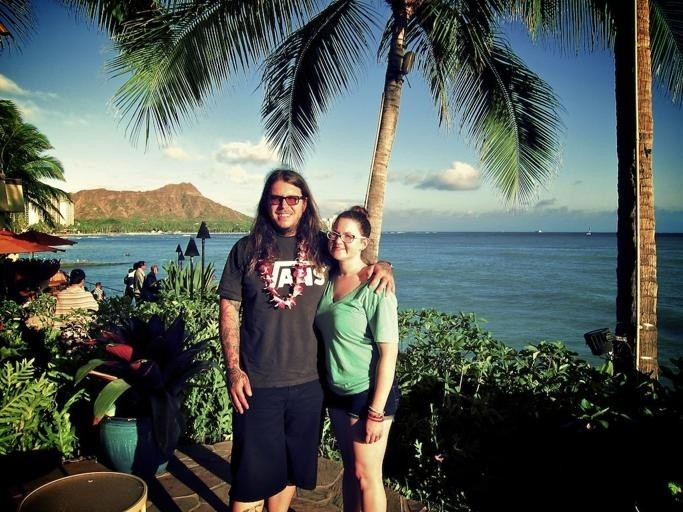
0 230 65 254
19 229 78 259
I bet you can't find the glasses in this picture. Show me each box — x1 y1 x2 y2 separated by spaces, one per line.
269 193 305 206
328 231 366 243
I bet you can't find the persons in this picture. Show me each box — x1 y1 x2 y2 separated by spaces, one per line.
215 169 397 512
92 282 107 304
124 260 161 307
53 269 99 314
314 206 400 512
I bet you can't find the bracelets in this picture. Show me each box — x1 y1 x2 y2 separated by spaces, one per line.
367 407 386 422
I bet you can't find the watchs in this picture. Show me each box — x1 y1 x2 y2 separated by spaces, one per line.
377 260 392 268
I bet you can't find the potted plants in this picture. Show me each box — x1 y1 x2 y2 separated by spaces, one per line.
74 306 220 478
